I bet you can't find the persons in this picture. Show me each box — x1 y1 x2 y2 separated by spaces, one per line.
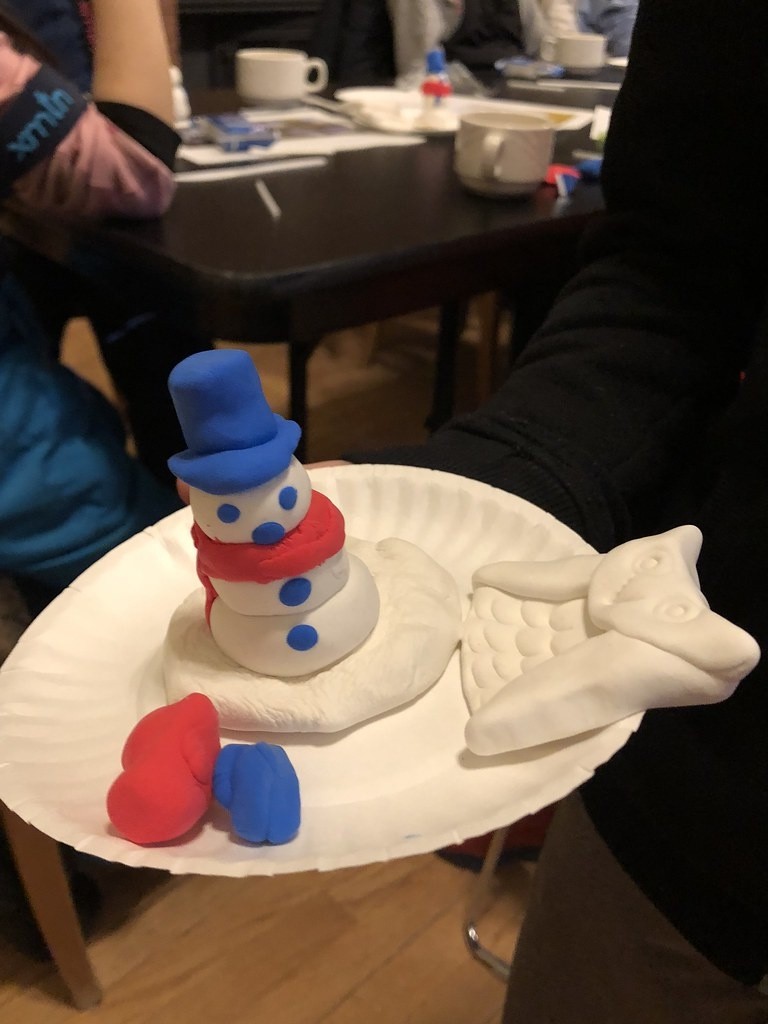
0 0 194 962
177 0 768 1024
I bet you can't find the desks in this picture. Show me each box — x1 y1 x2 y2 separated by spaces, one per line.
92 58 631 463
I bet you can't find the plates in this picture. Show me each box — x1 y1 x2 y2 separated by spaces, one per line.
336 87 472 133
0 463 647 878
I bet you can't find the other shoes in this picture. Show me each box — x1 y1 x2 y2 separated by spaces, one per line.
1 869 102 959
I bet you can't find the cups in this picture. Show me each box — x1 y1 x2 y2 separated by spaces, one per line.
235 47 330 106
539 32 607 71
453 112 555 197
